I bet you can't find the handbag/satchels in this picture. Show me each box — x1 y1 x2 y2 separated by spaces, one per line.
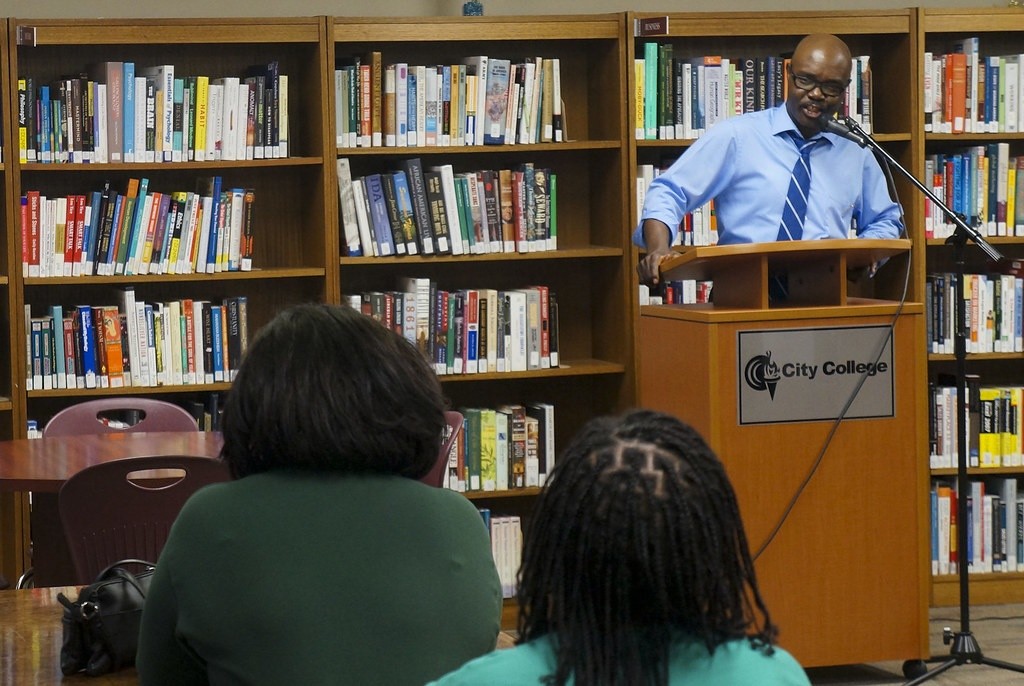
57 558 157 677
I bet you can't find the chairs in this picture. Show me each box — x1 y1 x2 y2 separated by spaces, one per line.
17 398 198 591
59 455 234 584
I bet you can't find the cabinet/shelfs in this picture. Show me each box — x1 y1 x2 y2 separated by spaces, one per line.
328 12 637 631
628 9 919 350
919 6 1024 607
9 16 334 433
0 17 22 590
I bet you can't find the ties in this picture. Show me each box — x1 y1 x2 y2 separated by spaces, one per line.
768 130 828 302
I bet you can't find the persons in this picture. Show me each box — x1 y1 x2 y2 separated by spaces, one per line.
137 299 506 686
422 406 813 686
632 33 904 306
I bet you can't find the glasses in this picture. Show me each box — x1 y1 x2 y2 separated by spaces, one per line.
789 66 851 97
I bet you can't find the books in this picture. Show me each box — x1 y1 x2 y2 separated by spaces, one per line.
633 41 877 304
8 32 570 599
925 35 1024 576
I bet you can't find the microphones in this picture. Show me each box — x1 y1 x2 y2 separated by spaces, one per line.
818 113 866 149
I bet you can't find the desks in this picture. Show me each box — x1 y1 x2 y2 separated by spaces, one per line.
2 585 141 686
0 431 225 494
